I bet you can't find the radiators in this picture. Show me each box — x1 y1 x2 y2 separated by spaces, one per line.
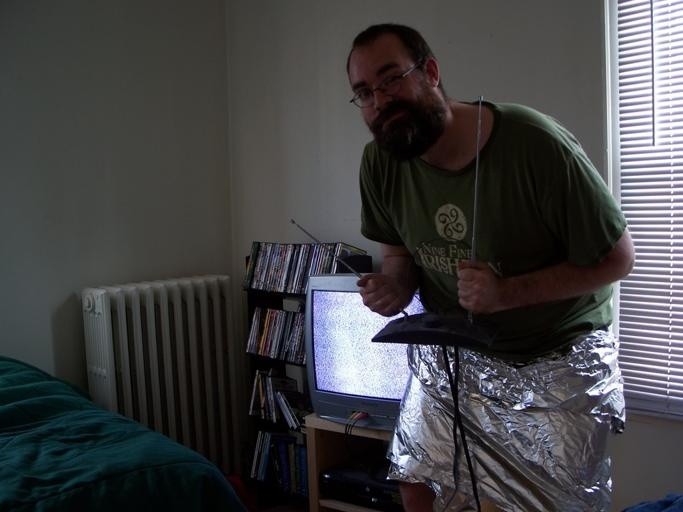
79 273 244 484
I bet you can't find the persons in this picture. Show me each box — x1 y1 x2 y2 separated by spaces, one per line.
346 24 637 512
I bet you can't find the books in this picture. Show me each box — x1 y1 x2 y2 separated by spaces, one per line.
234 234 367 494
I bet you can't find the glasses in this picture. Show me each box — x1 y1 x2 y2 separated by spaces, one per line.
349 62 422 108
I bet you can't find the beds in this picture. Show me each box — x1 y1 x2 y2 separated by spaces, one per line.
0 353 248 512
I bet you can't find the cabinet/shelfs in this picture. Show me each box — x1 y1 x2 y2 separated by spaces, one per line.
241 241 373 499
299 413 404 512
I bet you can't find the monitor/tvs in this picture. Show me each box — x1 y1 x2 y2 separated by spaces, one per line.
303 272 426 431
